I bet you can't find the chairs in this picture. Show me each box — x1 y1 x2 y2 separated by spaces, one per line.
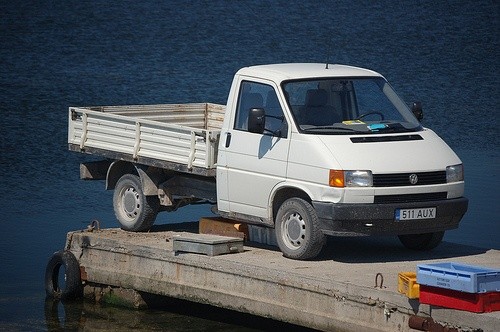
245 89 339 136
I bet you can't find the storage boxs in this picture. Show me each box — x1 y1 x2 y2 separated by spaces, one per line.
248 225 278 246
415 261 500 293
419 284 500 313
398 272 419 298
199 218 247 234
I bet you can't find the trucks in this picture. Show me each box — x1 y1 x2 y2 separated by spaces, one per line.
68 63 468 260
